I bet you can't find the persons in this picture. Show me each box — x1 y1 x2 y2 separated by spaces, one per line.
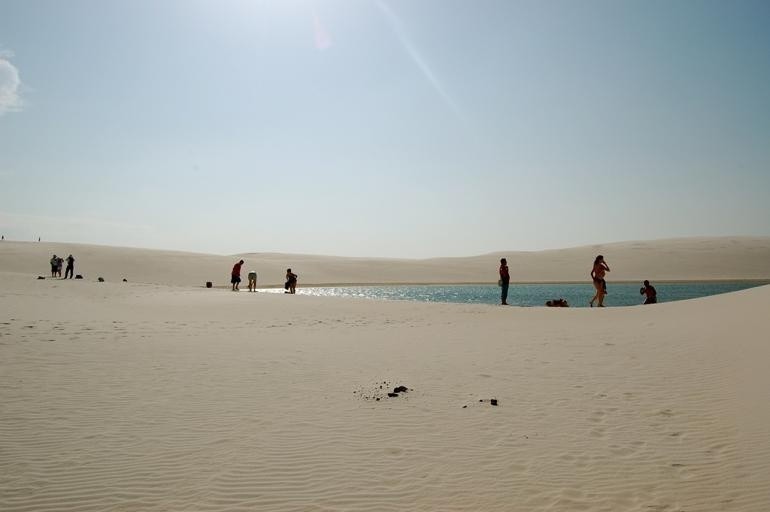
50 255 59 278
63 255 75 279
588 255 611 307
640 280 657 304
499 256 511 306
247 270 258 292
285 268 298 294
56 257 63 277
230 259 244 291
